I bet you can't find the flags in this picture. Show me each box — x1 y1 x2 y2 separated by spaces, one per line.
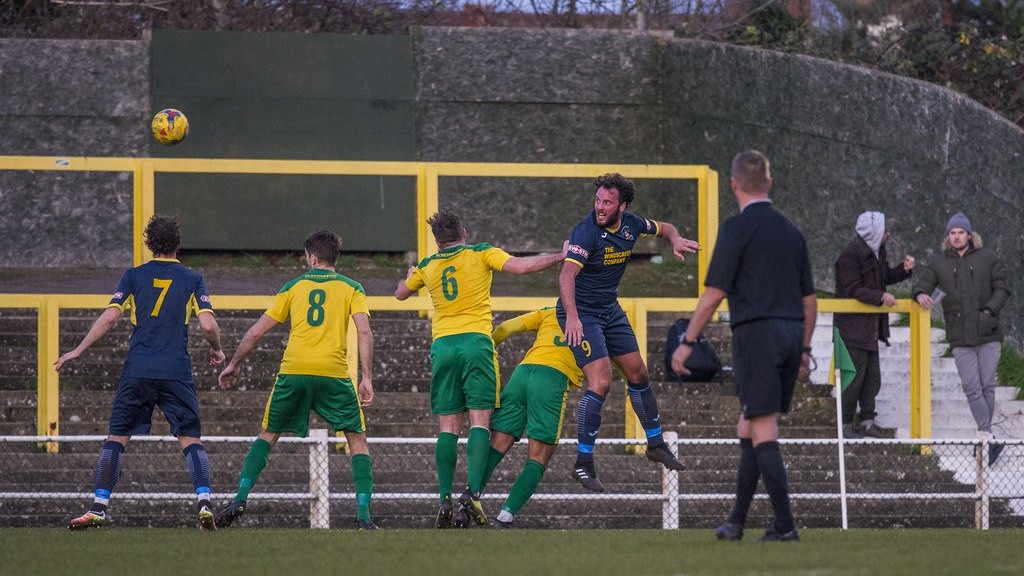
827 328 857 399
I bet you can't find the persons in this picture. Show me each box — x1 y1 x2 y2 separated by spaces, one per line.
54 215 225 531
671 149 818 543
214 230 382 530
833 211 915 438
395 210 570 528
556 173 702 493
911 213 1012 469
451 307 628 529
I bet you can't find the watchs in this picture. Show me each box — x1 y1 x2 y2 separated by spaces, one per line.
679 332 698 347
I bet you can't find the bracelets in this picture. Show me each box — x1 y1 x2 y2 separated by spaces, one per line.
802 346 812 353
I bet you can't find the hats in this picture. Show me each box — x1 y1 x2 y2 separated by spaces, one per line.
946 212 971 234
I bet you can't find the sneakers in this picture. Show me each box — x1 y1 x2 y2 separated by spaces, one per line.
355 519 380 532
750 524 799 541
216 501 246 529
458 489 488 529
453 508 470 530
197 504 217 531
492 518 515 530
435 495 453 529
571 461 604 492
987 443 1005 468
68 510 106 530
844 426 863 438
646 442 686 471
859 423 893 439
716 521 744 542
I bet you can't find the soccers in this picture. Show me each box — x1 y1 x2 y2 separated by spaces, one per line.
151 108 190 146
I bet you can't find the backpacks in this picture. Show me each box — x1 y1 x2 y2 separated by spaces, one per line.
663 318 723 386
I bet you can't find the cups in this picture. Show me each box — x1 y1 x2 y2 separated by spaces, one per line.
931 287 945 307
